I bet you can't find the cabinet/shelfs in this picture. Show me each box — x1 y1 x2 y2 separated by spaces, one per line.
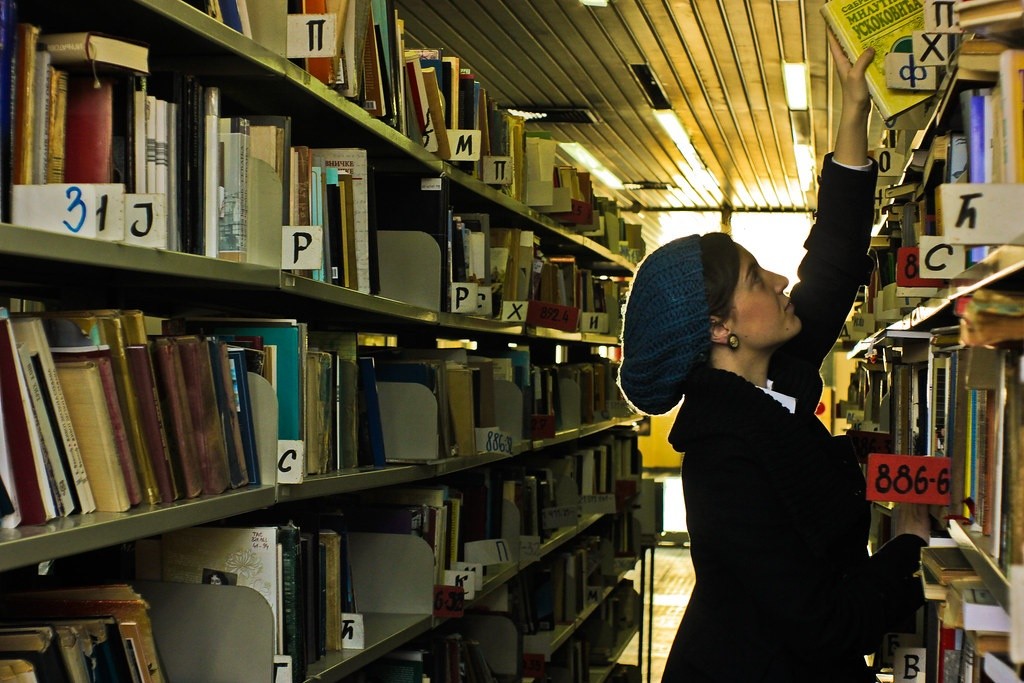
842 0 1024 683
0 0 652 683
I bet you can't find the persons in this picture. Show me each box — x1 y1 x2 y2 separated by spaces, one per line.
618 26 929 683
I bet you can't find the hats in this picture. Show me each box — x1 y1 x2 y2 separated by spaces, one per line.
619 235 709 414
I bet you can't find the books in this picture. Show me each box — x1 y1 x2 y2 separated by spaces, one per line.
0 0 1024 683
